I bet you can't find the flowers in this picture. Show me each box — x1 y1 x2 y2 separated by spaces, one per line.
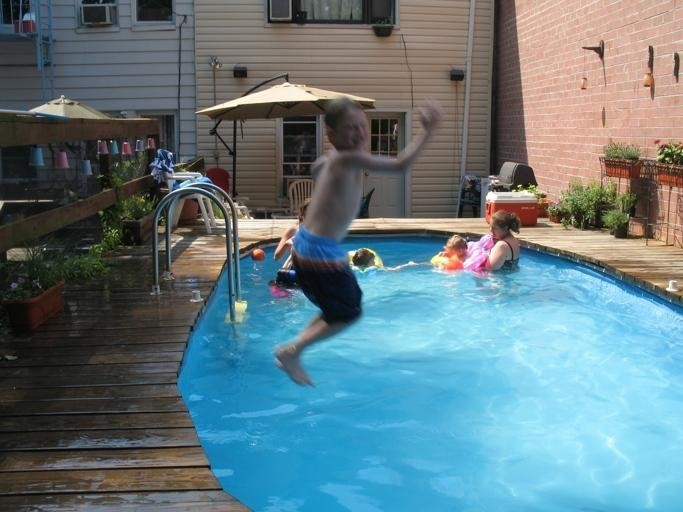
0 222 63 301
654 139 683 166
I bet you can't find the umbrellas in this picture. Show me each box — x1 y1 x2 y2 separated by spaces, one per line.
195 73 376 202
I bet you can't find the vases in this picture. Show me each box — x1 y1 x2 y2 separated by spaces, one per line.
0 275 66 331
654 163 683 188
159 186 200 221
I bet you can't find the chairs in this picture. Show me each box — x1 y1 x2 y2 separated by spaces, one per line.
288 178 317 216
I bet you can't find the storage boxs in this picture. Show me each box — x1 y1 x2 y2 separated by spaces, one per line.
485 191 538 227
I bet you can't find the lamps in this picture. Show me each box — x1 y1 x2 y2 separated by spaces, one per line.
581 39 605 89
643 45 654 87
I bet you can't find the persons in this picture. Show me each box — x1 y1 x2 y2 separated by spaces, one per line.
439 235 468 258
274 96 443 386
472 210 520 307
274 200 311 270
352 249 376 271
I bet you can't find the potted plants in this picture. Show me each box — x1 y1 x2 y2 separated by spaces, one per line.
604 142 641 178
549 175 639 239
98 193 156 245
372 16 394 37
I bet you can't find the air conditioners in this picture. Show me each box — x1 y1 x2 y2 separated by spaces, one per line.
79 4 116 26
